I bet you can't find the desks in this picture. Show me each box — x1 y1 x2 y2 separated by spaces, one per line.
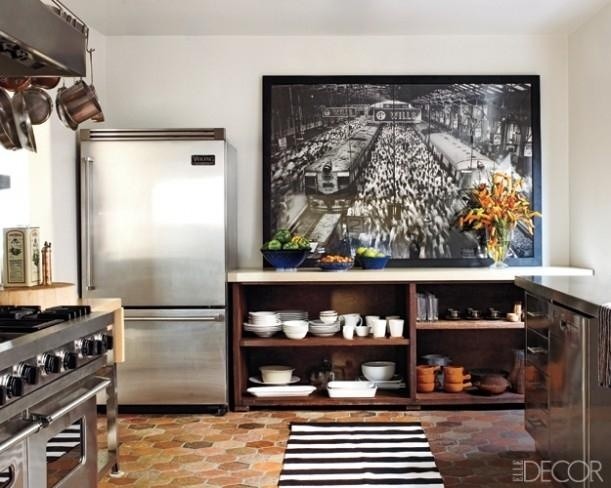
0 279 129 366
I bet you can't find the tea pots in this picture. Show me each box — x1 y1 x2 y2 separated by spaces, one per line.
306 358 337 394
510 348 526 395
422 353 450 390
470 373 512 395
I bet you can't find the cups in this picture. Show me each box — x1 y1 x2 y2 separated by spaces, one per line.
341 312 404 340
418 291 526 322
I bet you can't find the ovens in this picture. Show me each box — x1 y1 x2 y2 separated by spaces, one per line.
0 354 114 487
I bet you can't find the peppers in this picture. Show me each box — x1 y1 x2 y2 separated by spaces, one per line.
262 228 310 250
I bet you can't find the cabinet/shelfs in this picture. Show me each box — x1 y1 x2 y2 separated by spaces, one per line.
516 289 591 487
224 267 413 412
413 266 594 409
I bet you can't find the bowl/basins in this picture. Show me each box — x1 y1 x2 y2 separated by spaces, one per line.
361 361 398 381
356 254 392 270
259 365 295 383
528 361 565 395
283 319 309 341
321 309 338 325
468 368 510 382
417 364 472 393
260 248 313 271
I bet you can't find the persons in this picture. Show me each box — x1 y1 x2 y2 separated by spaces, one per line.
271 111 491 257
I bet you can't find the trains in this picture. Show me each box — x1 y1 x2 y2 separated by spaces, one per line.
411 120 500 195
305 121 387 214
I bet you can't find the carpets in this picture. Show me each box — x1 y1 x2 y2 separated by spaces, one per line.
273 419 447 488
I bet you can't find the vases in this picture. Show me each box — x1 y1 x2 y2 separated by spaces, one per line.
483 221 511 270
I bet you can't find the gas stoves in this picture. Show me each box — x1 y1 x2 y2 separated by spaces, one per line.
0 294 116 408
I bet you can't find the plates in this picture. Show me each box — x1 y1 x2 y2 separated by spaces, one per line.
310 320 338 338
249 376 301 386
245 310 307 338
246 383 317 398
318 262 353 271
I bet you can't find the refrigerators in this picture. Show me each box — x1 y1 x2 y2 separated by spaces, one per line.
80 126 239 418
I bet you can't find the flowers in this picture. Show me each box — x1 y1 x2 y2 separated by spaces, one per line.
452 166 541 261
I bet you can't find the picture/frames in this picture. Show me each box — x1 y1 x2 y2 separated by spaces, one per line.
260 74 544 268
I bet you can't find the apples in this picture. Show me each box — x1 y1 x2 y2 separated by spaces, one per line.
355 247 385 258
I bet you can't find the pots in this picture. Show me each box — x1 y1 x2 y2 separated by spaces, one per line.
0 51 107 154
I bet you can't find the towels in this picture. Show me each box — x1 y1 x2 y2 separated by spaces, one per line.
594 300 611 390
